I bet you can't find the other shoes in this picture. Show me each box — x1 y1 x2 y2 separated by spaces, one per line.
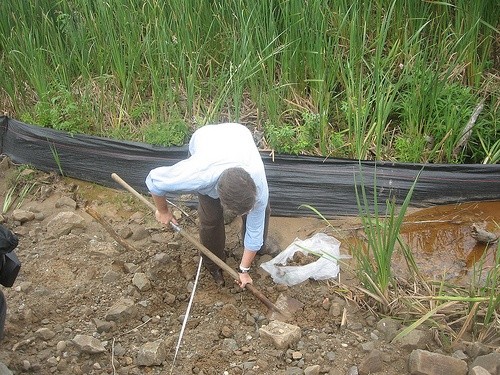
258 249 264 255
213 269 225 286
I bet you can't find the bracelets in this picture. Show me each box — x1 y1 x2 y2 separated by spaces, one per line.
238 265 250 273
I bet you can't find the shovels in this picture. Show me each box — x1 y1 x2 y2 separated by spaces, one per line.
111 172 305 326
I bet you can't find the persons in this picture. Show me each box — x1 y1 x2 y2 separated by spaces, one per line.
144 122 271 290
